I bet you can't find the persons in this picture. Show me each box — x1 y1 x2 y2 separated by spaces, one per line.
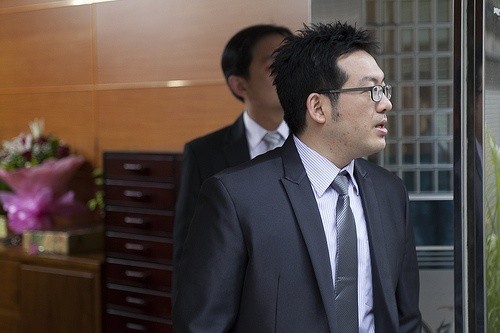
170 21 423 333
170 23 295 272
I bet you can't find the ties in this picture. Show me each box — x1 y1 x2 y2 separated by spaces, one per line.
329 174 359 333
262 133 281 152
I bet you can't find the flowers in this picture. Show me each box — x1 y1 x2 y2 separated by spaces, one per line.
0 116 105 235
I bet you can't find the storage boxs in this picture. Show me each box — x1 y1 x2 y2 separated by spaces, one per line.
21 223 103 256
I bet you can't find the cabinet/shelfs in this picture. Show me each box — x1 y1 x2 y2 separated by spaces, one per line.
103 152 183 333
0 246 105 333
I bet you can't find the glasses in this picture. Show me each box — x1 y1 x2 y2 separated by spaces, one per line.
318 84 393 103
